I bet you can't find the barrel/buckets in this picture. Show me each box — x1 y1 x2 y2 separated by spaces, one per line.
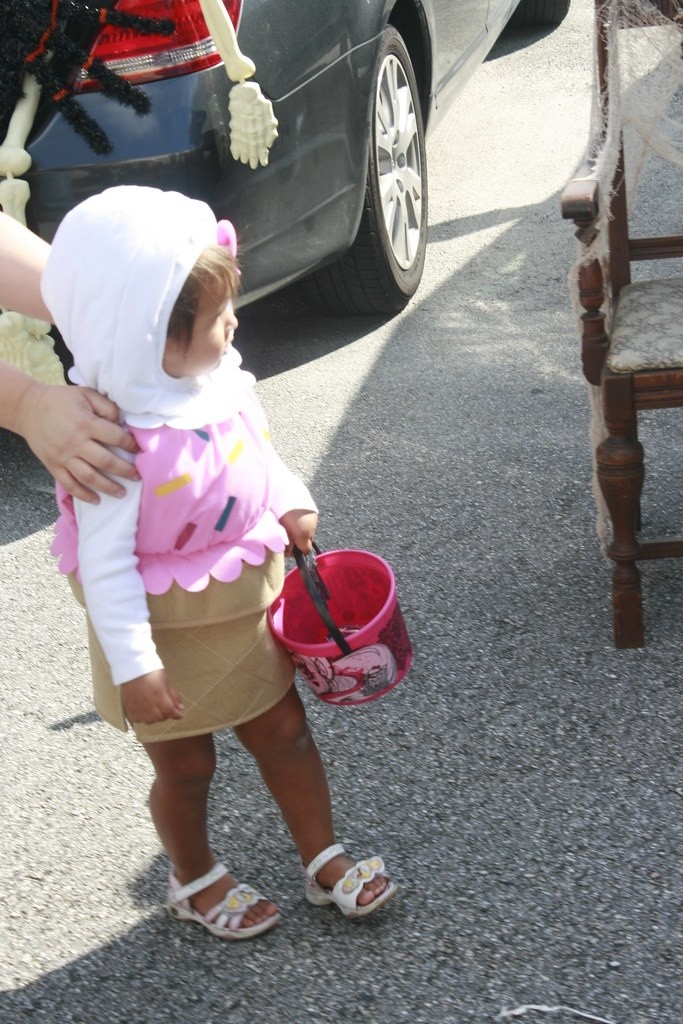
263 537 412 705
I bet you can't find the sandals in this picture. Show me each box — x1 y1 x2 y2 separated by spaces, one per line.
166 865 281 942
303 844 398 919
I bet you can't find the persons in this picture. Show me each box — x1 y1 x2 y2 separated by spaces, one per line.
0 208 141 505
45 181 397 941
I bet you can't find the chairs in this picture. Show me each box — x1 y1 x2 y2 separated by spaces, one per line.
560 0 683 649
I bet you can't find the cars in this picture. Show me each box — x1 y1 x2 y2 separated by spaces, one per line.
1 0 573 321
